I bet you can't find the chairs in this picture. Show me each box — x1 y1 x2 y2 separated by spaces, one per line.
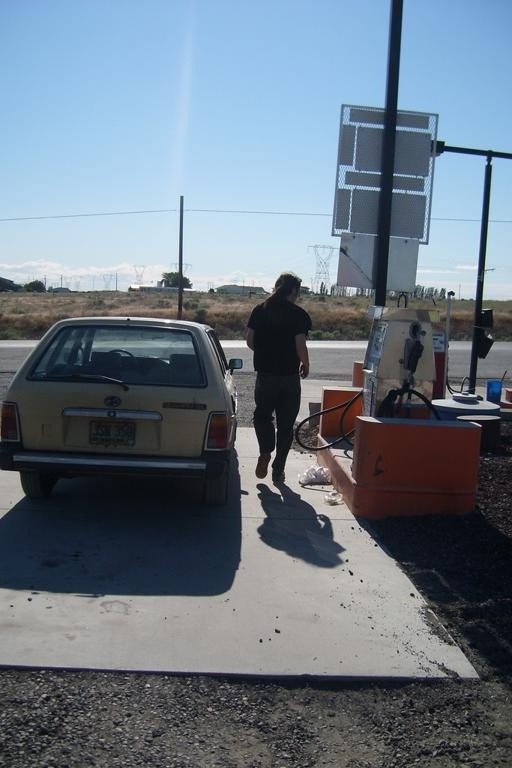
48 351 196 384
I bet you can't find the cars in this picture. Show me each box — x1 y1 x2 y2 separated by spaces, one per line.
0 316 243 506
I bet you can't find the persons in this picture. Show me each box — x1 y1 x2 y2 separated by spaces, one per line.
246 273 313 483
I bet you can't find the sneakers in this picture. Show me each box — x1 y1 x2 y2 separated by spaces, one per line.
255 452 272 479
271 470 287 484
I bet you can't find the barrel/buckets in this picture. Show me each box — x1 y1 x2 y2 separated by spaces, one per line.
486 380 502 402
